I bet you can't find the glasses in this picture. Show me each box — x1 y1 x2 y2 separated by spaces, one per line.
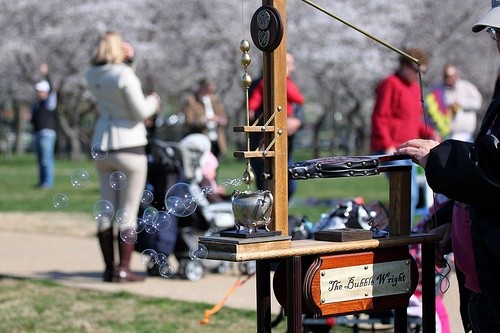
411 65 426 75
486 27 500 41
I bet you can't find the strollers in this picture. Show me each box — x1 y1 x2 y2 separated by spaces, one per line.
133 137 257 282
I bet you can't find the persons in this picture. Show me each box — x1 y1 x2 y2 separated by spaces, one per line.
29 63 60 191
85 29 163 284
244 50 305 201
393 0 500 333
425 62 483 142
184 77 228 159
194 151 230 204
368 48 442 227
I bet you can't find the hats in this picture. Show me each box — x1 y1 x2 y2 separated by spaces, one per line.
471 5 500 33
35 80 50 92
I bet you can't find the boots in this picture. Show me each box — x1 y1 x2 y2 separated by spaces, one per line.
97 226 115 281
116 228 144 282
